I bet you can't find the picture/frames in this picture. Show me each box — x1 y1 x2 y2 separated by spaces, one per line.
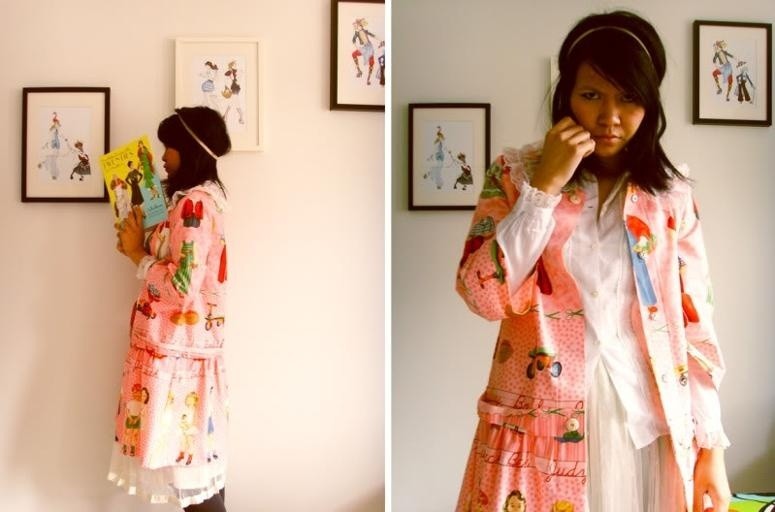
692 19 773 127
329 0 386 113
173 37 265 152
407 103 491 210
21 86 110 203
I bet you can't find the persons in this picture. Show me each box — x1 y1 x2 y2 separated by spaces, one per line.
38 113 67 181
64 138 92 182
200 60 220 112
137 140 161 202
734 58 757 102
449 151 474 191
350 19 379 85
111 173 130 220
219 61 245 126
421 126 453 191
124 161 145 218
377 41 386 85
452 9 738 511
710 39 738 101
105 106 234 511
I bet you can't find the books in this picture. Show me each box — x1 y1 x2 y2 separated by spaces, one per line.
96 133 169 235
